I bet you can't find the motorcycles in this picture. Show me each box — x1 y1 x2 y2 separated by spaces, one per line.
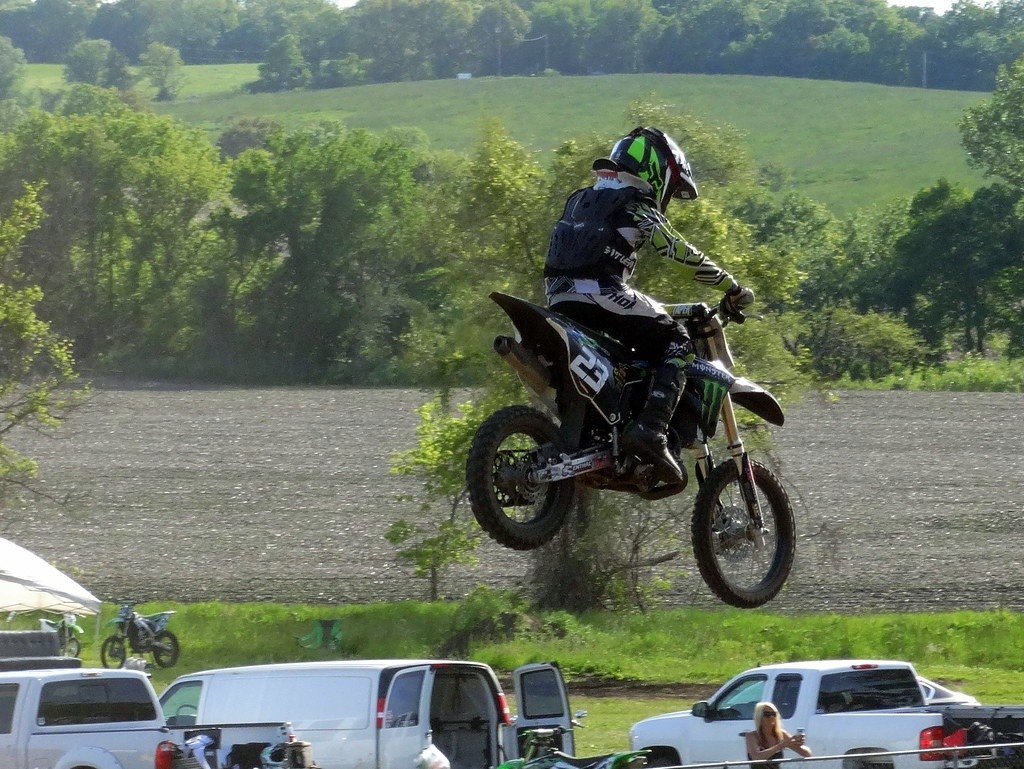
34 612 85 658
100 600 180 670
497 726 653 769
463 292 797 610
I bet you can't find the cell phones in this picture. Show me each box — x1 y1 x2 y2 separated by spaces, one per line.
797 728 805 738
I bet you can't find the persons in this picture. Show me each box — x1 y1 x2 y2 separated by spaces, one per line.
543 125 756 485
746 701 811 769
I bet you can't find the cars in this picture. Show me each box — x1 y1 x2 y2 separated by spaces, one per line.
725 676 981 706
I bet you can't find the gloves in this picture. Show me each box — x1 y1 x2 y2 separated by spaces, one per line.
717 285 754 324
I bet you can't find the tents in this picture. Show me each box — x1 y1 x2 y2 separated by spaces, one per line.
0 536 104 621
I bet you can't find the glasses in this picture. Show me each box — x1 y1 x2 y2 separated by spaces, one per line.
763 711 776 717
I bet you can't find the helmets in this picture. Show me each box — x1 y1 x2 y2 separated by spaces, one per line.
592 126 698 200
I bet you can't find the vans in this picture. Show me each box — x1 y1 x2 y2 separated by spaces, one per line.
158 659 576 769
0 669 174 769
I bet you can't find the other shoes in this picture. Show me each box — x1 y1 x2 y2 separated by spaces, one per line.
623 421 683 484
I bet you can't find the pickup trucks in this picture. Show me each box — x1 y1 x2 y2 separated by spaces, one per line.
629 660 1024 769
